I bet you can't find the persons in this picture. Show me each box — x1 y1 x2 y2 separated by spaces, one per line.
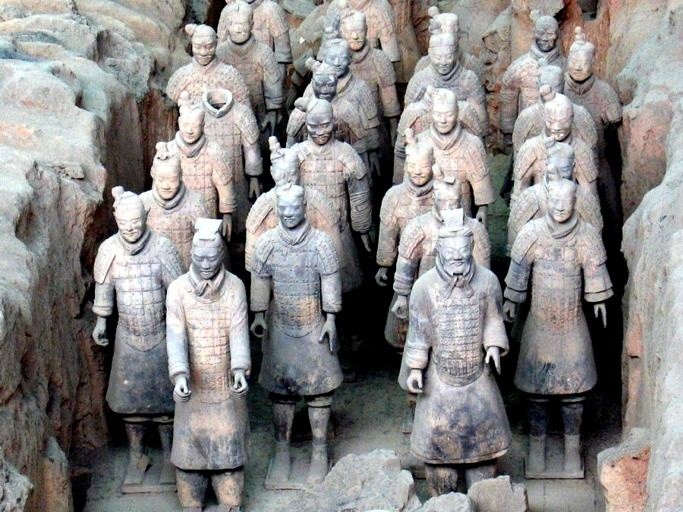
89 0 628 511
